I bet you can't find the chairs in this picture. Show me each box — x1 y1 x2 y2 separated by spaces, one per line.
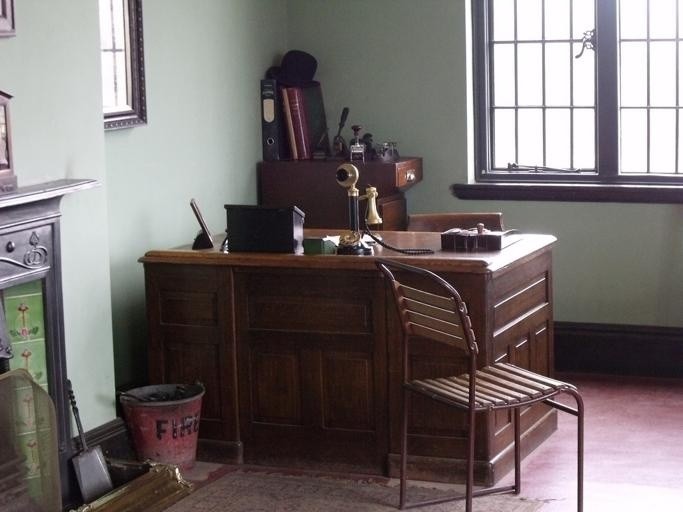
407 212 504 232
375 259 584 512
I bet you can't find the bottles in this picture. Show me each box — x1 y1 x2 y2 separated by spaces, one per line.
349 125 364 160
376 141 398 161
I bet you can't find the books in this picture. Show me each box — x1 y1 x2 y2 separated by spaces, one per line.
259 79 331 162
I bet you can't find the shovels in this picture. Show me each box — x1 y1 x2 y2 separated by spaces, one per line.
66 380 114 505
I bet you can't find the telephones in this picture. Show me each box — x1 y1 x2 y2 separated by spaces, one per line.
335 163 383 255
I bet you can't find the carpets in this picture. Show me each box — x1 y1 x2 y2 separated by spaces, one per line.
162 468 557 512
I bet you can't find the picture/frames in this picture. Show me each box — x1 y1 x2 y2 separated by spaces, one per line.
0 0 16 37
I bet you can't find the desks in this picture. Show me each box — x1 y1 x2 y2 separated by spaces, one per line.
137 228 558 487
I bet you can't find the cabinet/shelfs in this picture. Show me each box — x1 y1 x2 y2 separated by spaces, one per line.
257 157 423 231
